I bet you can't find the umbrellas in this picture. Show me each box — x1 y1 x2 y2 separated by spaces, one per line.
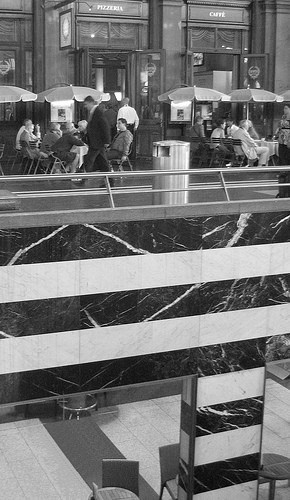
0 85 38 124
275 90 290 101
33 85 111 121
227 88 284 120
157 85 231 126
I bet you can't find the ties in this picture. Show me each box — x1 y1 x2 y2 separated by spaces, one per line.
107 131 125 149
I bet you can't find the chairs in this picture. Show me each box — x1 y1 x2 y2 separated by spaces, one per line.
0 140 134 180
101 458 139 497
182 135 280 167
159 443 179 500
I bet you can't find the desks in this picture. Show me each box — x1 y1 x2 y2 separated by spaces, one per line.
255 139 279 166
258 453 290 500
70 144 89 172
88 487 139 500
57 394 97 421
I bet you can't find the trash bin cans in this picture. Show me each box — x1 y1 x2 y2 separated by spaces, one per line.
153 140 190 205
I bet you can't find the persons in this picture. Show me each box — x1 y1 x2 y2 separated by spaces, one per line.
273 172 290 198
74 96 115 188
272 104 290 178
15 116 269 181
116 97 140 166
101 102 117 141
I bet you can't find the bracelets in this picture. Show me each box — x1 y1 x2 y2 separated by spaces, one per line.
274 133 277 136
